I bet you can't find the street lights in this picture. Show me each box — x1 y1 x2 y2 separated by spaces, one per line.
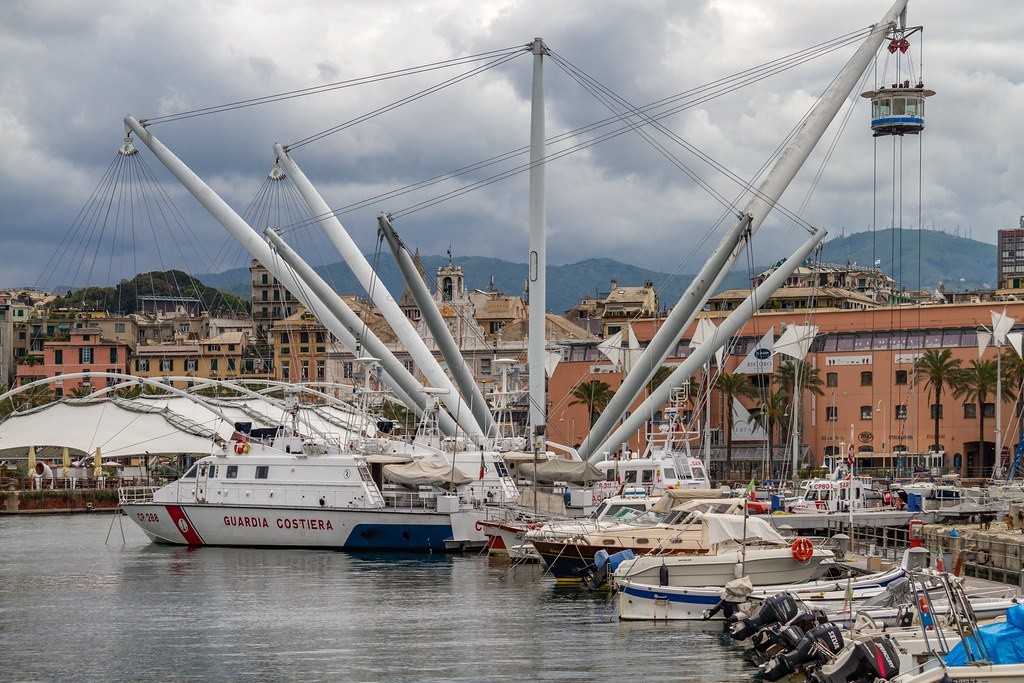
560 411 575 448
876 400 886 477
760 403 770 481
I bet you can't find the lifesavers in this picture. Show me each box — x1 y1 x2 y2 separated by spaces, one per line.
792 537 813 562
597 495 601 501
573 535 591 546
920 598 934 630
475 520 482 531
895 497 903 510
234 438 245 454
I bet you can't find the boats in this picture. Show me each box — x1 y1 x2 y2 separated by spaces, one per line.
475 488 793 585
104 437 519 547
611 534 1024 683
